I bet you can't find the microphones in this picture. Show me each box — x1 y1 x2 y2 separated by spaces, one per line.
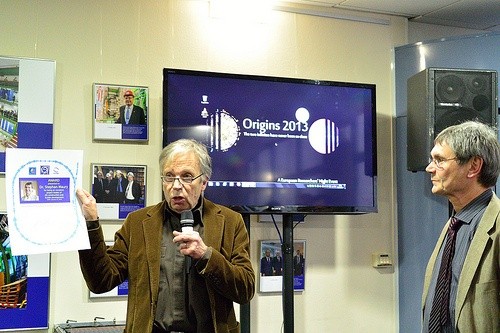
181 210 195 272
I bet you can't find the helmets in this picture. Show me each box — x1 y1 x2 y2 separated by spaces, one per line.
124 91 135 97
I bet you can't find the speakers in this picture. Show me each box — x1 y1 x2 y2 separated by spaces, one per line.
406 66 497 173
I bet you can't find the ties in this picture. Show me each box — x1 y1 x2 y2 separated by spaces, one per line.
118 177 120 193
427 218 462 333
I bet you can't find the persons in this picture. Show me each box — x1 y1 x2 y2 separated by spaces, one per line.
420 120 500 333
21 181 40 202
74 139 254 332
260 249 305 277
114 91 146 127
92 169 143 205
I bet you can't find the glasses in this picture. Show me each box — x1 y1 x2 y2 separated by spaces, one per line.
428 157 459 166
161 173 203 185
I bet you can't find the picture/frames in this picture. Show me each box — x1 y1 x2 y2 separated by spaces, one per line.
92 82 149 145
89 162 148 225
88 239 129 303
258 240 307 294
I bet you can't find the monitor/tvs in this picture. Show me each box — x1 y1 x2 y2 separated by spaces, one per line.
162 67 378 213
